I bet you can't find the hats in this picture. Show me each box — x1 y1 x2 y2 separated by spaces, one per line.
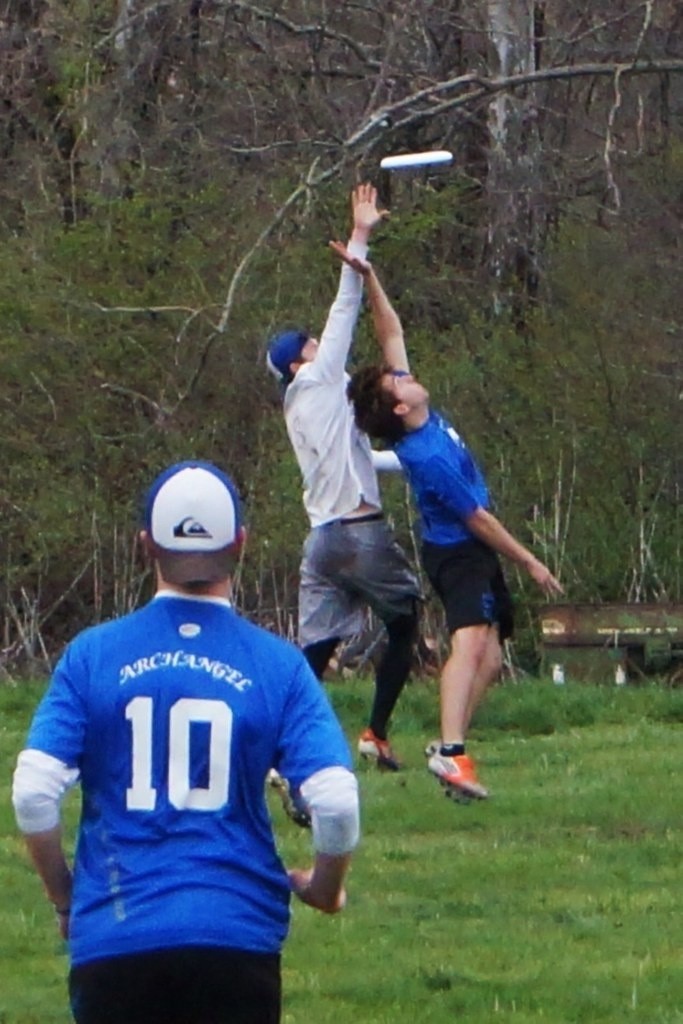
146 460 240 585
265 331 307 400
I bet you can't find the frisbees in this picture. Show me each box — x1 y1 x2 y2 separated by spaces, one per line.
380 150 453 169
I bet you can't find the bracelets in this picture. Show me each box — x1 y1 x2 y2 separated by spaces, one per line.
54 905 70 917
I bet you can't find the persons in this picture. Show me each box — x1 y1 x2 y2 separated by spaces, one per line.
327 238 567 803
266 182 425 771
12 459 360 1024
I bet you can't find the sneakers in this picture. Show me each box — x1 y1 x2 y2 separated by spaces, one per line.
357 728 398 771
425 743 473 805
427 746 489 798
268 768 312 828
